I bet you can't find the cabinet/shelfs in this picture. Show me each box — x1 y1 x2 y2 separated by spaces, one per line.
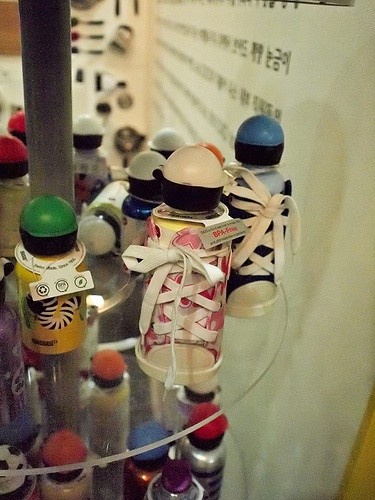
0 0 292 474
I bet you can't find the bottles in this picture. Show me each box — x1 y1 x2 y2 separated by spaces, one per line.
2 104 301 500
